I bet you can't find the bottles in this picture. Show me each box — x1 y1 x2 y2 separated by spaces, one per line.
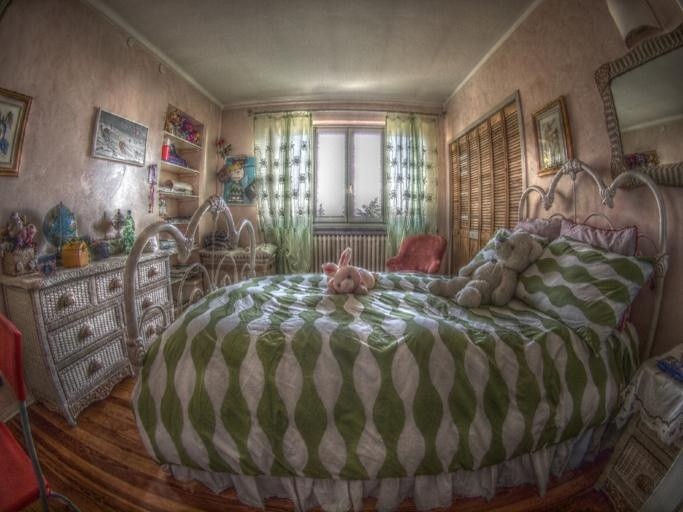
123 209 136 254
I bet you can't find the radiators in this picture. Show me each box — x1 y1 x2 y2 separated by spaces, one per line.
312 234 386 274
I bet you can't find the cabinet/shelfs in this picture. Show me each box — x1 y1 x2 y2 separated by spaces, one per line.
158 103 208 305
202 245 278 294
0 248 175 428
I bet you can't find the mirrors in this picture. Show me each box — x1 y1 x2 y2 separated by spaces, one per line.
595 21 683 190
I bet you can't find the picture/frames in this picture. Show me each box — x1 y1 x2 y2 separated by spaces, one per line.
530 95 574 177
0 87 34 177
90 106 149 168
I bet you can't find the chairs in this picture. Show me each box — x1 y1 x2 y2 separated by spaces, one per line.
385 234 447 274
0 310 51 512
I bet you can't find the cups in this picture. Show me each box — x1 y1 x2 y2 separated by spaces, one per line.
34 252 57 278
162 144 170 161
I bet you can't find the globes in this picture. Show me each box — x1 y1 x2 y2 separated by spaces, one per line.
43 201 77 266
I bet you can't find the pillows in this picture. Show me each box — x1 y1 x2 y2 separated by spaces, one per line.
468 215 656 356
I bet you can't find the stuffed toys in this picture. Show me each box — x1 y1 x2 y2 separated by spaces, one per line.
426 229 543 307
321 251 375 298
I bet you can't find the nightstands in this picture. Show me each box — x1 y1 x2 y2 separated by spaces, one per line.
592 344 682 508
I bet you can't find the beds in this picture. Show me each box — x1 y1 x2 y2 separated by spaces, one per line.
130 158 670 511
